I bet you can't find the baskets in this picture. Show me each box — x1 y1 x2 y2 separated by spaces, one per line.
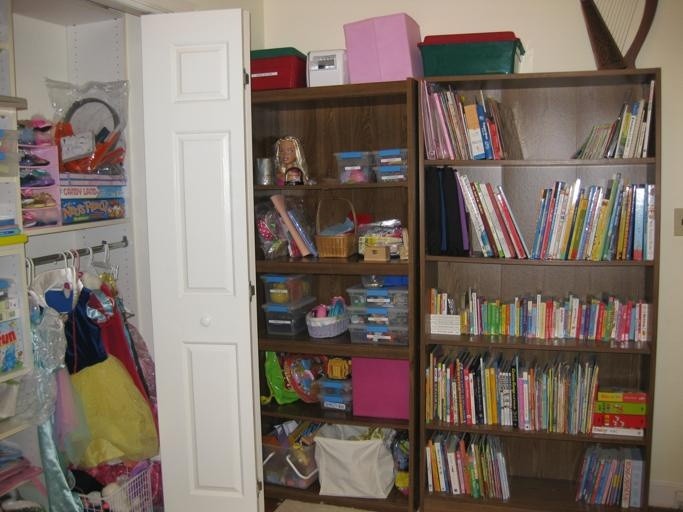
315 198 357 259
74 461 155 512
305 305 350 339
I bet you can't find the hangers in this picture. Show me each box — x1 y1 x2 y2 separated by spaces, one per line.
25 238 118 295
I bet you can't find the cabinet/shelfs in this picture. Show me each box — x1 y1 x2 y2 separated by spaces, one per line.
1 1 270 512
416 69 661 510
250 78 415 509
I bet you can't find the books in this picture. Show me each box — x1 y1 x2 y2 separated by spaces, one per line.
425 345 600 435
425 432 511 499
425 166 530 259
422 81 523 161
575 444 644 507
531 173 656 261
578 80 655 161
592 387 648 437
430 287 655 342
59 174 129 199
265 193 318 260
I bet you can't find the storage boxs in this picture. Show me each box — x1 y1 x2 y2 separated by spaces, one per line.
262 429 319 490
314 423 395 500
343 12 423 84
252 47 306 86
415 28 524 75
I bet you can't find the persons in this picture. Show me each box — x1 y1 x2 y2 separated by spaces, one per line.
272 134 315 185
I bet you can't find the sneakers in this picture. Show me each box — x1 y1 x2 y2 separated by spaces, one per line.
17 118 56 228
